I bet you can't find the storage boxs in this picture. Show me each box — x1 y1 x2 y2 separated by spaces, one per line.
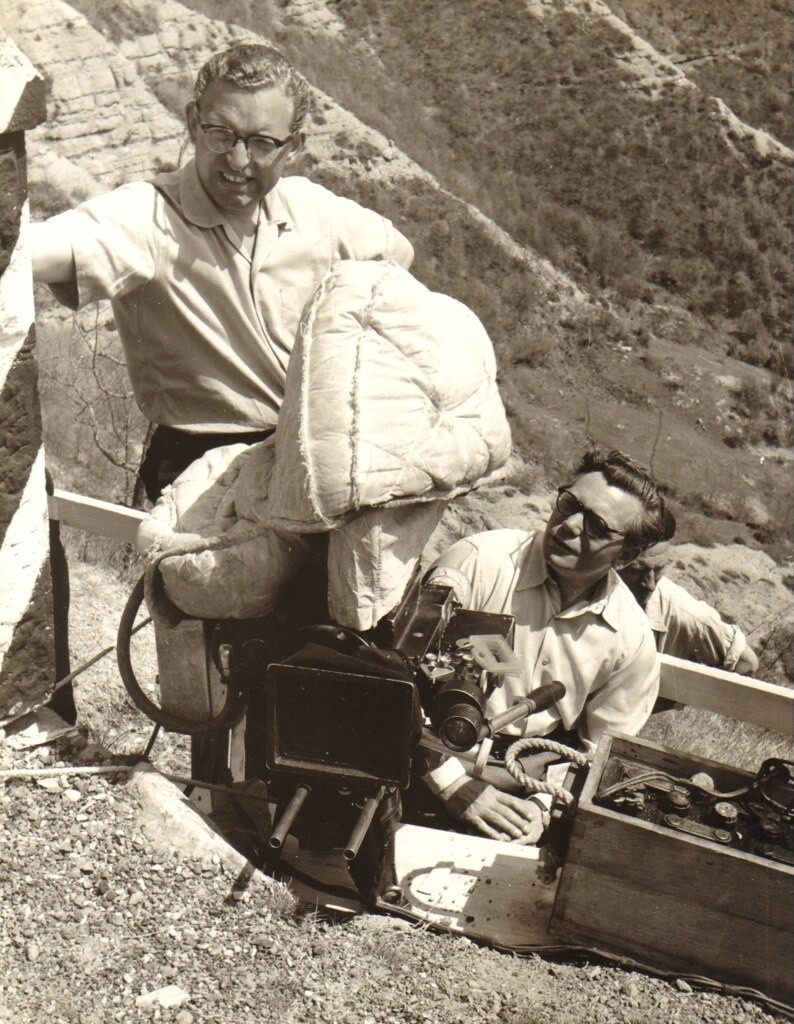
545 728 794 1008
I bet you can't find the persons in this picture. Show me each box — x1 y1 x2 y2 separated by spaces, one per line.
421 452 665 846
617 508 759 715
31 44 415 508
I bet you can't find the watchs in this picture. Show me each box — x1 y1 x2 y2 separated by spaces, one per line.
529 797 550 828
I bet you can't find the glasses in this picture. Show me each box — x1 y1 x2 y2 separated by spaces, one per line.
556 482 631 540
192 100 299 165
